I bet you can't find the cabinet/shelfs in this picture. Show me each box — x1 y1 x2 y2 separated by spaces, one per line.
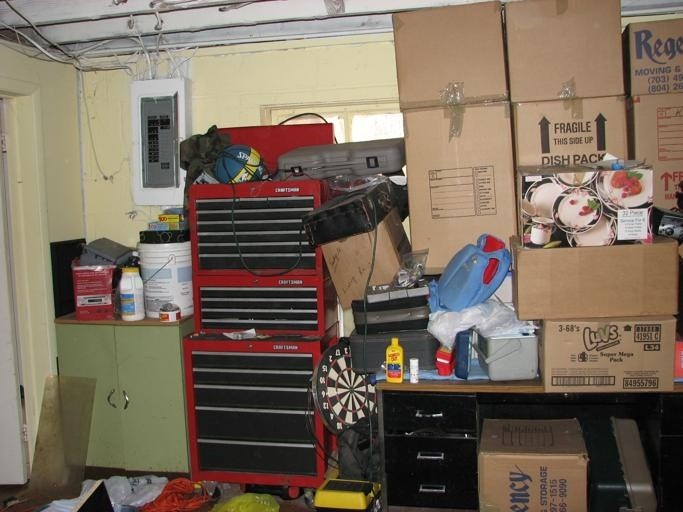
375 377 682 511
55 311 198 476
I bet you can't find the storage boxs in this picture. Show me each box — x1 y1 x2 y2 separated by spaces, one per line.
391 0 682 394
315 206 411 311
474 418 592 511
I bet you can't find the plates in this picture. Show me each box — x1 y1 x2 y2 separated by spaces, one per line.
525 170 654 247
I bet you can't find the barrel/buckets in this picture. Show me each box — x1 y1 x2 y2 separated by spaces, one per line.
120 266 145 321
136 241 195 319
